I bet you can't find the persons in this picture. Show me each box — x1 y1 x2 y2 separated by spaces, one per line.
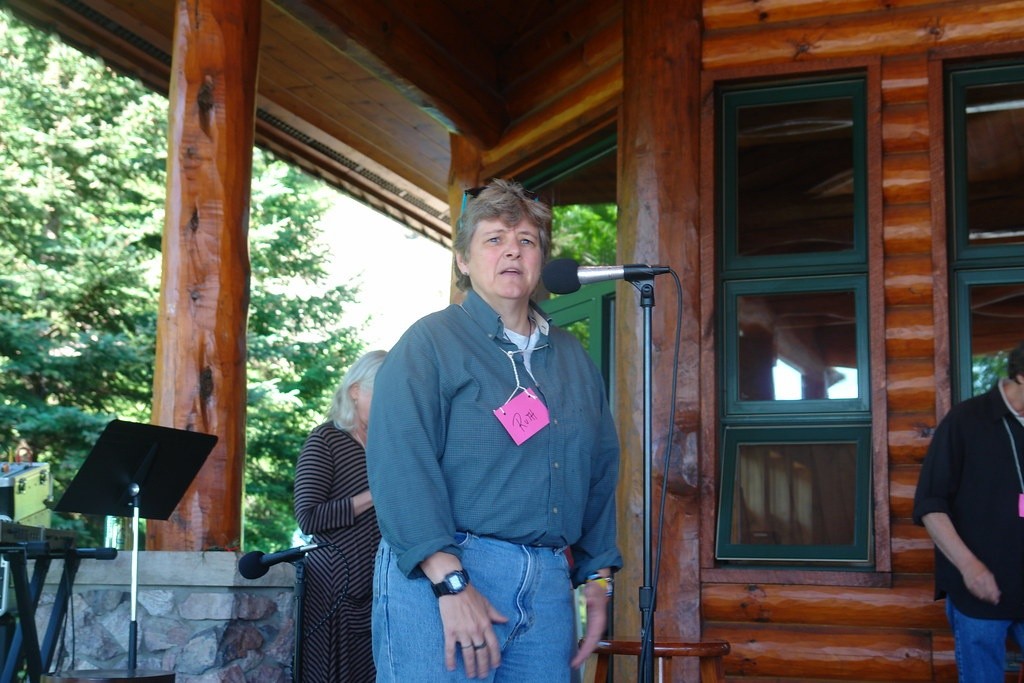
910 339 1023 683
366 178 623 683
292 349 388 683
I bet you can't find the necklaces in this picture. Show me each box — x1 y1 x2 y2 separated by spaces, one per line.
503 315 531 360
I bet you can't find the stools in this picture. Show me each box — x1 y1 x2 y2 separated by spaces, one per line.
576 638 732 683
40 670 176 683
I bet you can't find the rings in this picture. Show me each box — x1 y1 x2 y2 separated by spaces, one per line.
461 642 473 649
475 640 487 650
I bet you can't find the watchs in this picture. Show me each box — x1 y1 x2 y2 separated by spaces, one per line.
430 567 470 598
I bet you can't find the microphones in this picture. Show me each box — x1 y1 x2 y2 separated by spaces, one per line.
239 543 330 580
542 258 670 294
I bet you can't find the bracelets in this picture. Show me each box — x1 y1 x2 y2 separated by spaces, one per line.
583 571 615 597
